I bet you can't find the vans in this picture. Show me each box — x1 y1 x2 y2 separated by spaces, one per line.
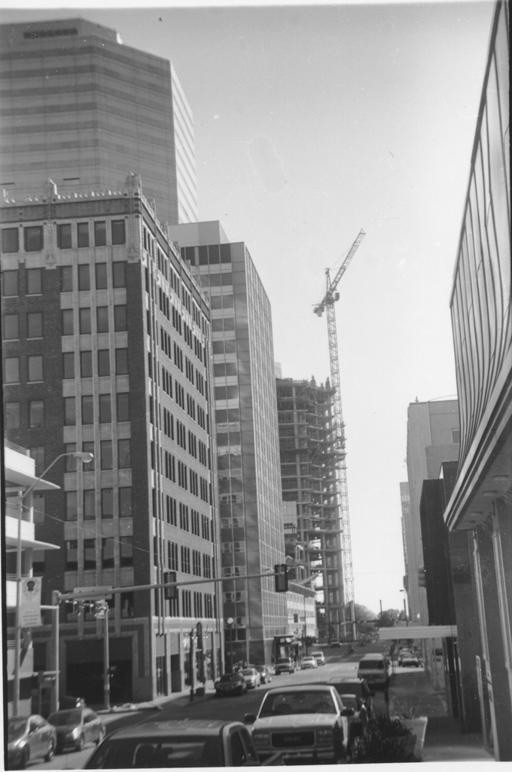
356 653 390 692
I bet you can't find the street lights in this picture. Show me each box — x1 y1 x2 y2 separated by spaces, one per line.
11 451 94 721
227 617 235 669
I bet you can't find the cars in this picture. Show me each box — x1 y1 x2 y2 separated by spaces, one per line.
213 665 273 698
6 706 108 769
274 657 296 676
300 651 326 670
242 684 369 767
397 647 420 668
81 719 265 769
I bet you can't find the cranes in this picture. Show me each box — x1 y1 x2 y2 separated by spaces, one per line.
311 229 368 607
